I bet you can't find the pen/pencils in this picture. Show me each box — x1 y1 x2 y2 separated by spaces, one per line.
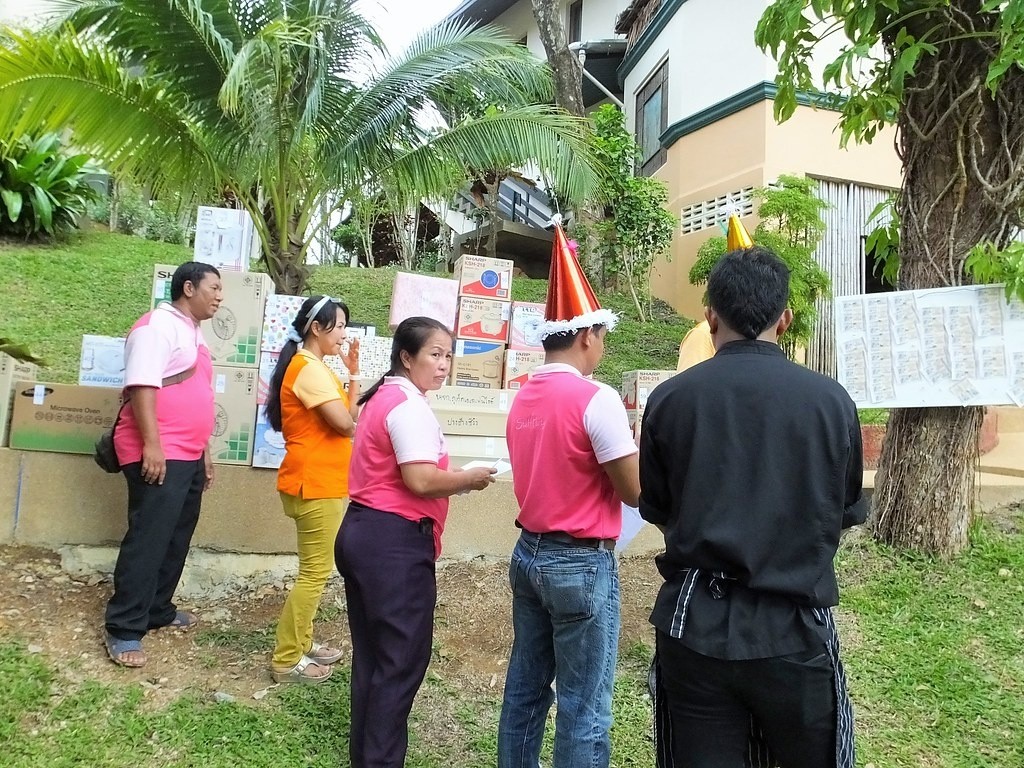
491 457 502 468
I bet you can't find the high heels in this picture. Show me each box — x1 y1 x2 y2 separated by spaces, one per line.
304 643 343 663
273 654 334 682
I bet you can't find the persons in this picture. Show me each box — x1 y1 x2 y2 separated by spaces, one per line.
640 247 866 768
266 294 361 683
498 220 642 767
334 316 497 767
100 261 223 668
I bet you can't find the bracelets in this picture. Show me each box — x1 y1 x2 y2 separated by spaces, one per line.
348 374 361 382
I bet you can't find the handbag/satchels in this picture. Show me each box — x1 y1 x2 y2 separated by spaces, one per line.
94 429 121 475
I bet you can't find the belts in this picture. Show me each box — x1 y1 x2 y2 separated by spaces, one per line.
515 519 616 551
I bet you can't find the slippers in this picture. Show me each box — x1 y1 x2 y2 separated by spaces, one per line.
166 611 195 629
105 631 146 667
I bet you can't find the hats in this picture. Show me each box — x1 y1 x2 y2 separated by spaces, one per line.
537 213 619 337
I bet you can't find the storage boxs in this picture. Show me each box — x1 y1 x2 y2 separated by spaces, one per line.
0 205 682 475
